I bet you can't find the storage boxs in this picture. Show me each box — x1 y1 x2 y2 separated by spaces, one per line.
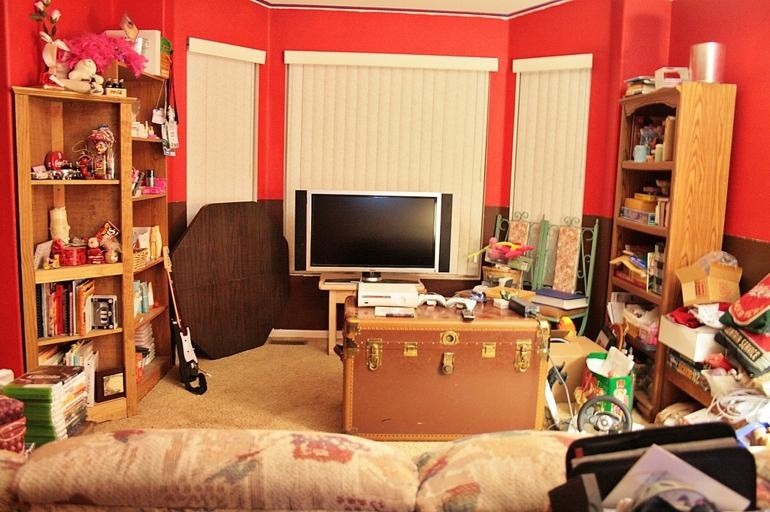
550 335 608 403
674 261 744 308
343 294 551 443
657 312 723 364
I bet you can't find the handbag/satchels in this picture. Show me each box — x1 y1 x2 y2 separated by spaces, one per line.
582 353 638 429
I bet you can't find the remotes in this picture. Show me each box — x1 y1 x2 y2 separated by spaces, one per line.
461 308 475 321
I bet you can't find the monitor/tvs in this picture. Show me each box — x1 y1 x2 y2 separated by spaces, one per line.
306 188 443 284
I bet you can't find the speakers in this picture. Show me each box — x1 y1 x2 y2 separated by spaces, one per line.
439 193 453 273
293 189 307 272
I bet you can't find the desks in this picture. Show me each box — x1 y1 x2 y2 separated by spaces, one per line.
327 290 428 356
661 363 728 413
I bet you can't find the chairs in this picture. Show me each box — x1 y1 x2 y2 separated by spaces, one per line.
534 218 601 336
494 212 544 291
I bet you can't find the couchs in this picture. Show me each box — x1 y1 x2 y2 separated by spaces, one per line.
1 429 770 512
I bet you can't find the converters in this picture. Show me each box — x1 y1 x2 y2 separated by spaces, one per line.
508 297 539 318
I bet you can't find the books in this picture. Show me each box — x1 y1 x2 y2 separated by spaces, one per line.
1 278 100 449
661 116 676 163
133 320 156 366
652 196 670 228
606 291 653 330
529 300 587 318
375 306 415 318
665 346 710 391
418 293 477 311
623 76 682 98
528 286 589 310
654 143 664 163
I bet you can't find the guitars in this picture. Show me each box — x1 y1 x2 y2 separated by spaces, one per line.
163 246 199 383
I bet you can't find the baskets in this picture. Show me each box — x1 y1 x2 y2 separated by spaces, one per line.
133 248 147 271
623 308 647 338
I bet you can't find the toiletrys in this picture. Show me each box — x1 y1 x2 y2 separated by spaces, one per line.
132 280 158 316
144 169 155 187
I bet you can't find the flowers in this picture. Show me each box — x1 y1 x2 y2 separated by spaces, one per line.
30 1 61 35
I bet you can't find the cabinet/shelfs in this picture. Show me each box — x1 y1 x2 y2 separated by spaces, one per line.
102 58 174 401
604 79 737 423
12 85 137 427
